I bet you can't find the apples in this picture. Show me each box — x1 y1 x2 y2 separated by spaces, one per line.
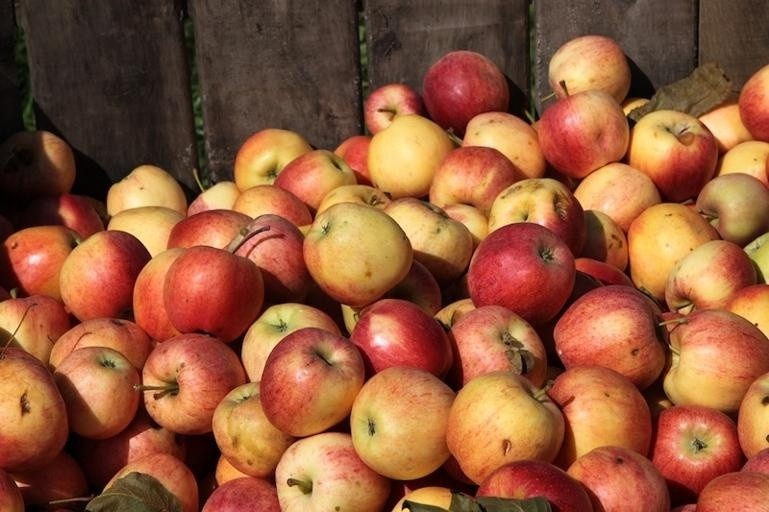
0 35 768 511
